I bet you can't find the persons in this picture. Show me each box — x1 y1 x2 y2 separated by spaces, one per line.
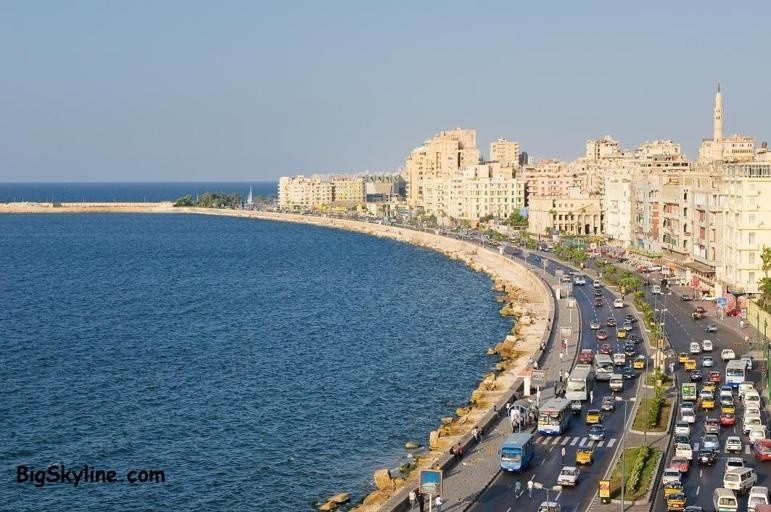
515 479 523 498
562 445 567 465
409 489 418 510
471 426 486 444
528 480 534 498
419 493 426 512
436 495 444 512
450 442 465 460
493 337 572 434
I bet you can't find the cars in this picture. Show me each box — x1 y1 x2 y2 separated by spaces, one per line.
497 265 771 512
434 226 631 266
